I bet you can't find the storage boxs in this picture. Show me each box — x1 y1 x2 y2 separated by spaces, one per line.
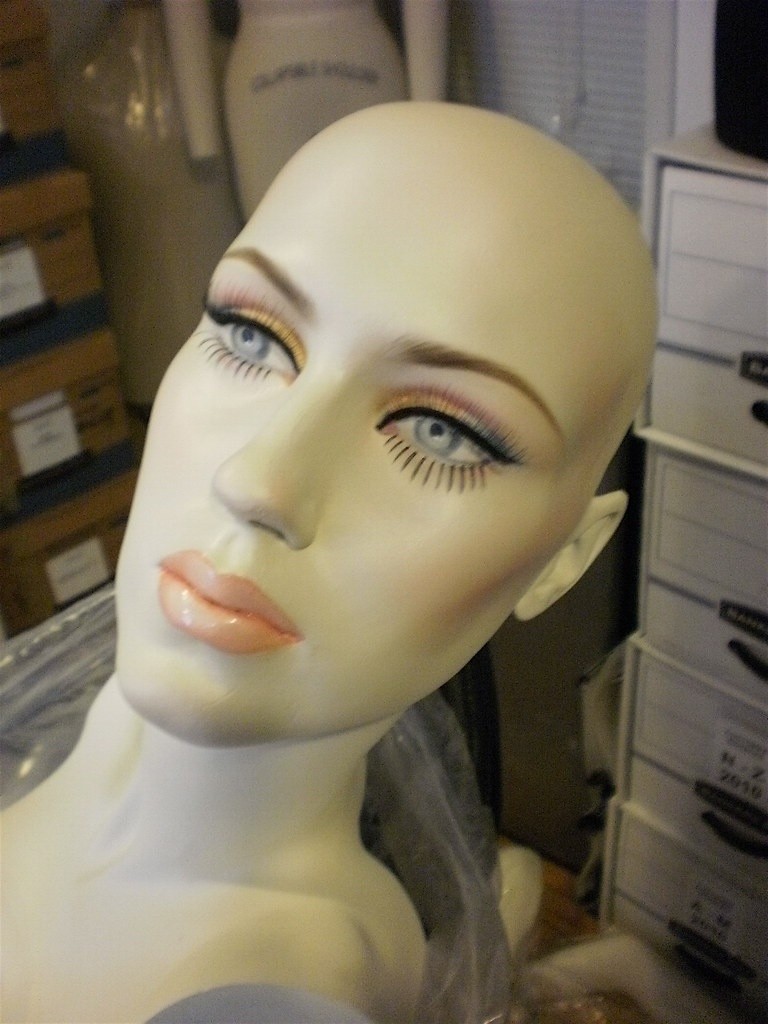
1 469 139 642
0 0 72 184
1 167 105 368
0 327 137 523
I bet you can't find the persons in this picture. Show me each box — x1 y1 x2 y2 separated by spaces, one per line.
0 99 661 1023
163 0 454 229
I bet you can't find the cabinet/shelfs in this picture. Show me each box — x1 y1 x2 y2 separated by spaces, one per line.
612 632 768 887
637 438 767 703
628 132 767 479
597 794 768 1024
